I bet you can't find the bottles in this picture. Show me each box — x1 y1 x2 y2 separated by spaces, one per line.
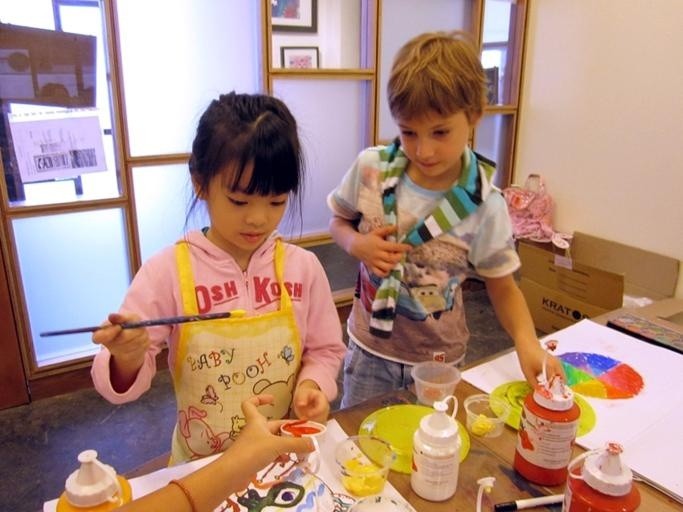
512 372 578 484
409 394 461 501
560 438 642 511
55 447 132 512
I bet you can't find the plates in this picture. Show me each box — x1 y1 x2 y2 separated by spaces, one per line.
491 382 595 437
359 405 470 474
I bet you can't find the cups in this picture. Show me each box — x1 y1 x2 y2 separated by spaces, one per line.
345 494 414 511
333 434 395 496
279 421 327 452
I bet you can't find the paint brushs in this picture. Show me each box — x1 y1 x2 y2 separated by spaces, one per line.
40 309 246 337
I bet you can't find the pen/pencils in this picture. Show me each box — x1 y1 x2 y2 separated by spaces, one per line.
494 494 565 512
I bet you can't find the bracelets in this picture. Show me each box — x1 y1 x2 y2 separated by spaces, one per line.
168 479 196 512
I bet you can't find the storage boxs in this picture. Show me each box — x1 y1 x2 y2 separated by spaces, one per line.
509 228 679 336
636 294 683 330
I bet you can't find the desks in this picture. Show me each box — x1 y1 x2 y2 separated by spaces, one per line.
315 302 682 512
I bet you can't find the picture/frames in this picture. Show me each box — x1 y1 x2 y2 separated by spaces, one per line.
278 46 320 70
269 0 317 35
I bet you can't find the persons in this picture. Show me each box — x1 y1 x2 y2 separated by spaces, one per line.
325 27 566 410
90 89 349 468
104 397 316 512
